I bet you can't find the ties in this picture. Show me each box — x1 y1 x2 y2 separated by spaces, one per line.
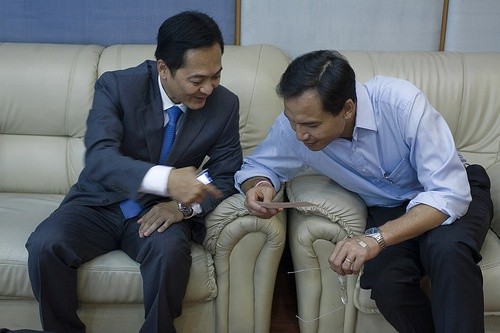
119 106 183 219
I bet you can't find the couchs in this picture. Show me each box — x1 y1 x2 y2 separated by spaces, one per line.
288 50 500 333
0 42 291 333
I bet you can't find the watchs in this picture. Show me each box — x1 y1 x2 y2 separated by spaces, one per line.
365 227 387 252
177 202 192 217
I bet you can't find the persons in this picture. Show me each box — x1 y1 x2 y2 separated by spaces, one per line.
234 50 493 333
25 8 243 333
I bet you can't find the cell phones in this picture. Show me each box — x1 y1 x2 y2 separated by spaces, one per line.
195 168 214 185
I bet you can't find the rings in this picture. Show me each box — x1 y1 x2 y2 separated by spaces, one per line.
345 258 352 264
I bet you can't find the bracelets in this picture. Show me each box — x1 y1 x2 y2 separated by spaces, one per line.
354 235 370 261
